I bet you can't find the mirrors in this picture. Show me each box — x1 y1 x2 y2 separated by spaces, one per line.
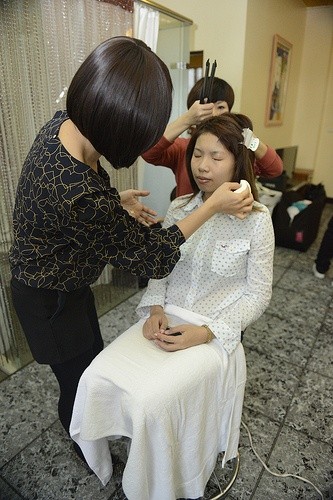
138 11 191 221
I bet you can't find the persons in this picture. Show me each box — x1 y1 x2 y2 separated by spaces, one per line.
10 36 254 500
312 217 333 280
70 111 273 500
141 76 284 199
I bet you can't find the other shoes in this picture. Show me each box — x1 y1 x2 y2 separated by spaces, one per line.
312 264 326 279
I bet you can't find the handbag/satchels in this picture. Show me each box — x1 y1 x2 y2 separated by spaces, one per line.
272 183 328 253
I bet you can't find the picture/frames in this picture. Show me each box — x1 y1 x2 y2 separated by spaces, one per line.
264 32 293 128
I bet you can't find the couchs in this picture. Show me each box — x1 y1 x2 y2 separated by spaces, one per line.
272 183 328 253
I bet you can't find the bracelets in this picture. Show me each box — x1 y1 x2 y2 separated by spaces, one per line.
201 324 212 344
